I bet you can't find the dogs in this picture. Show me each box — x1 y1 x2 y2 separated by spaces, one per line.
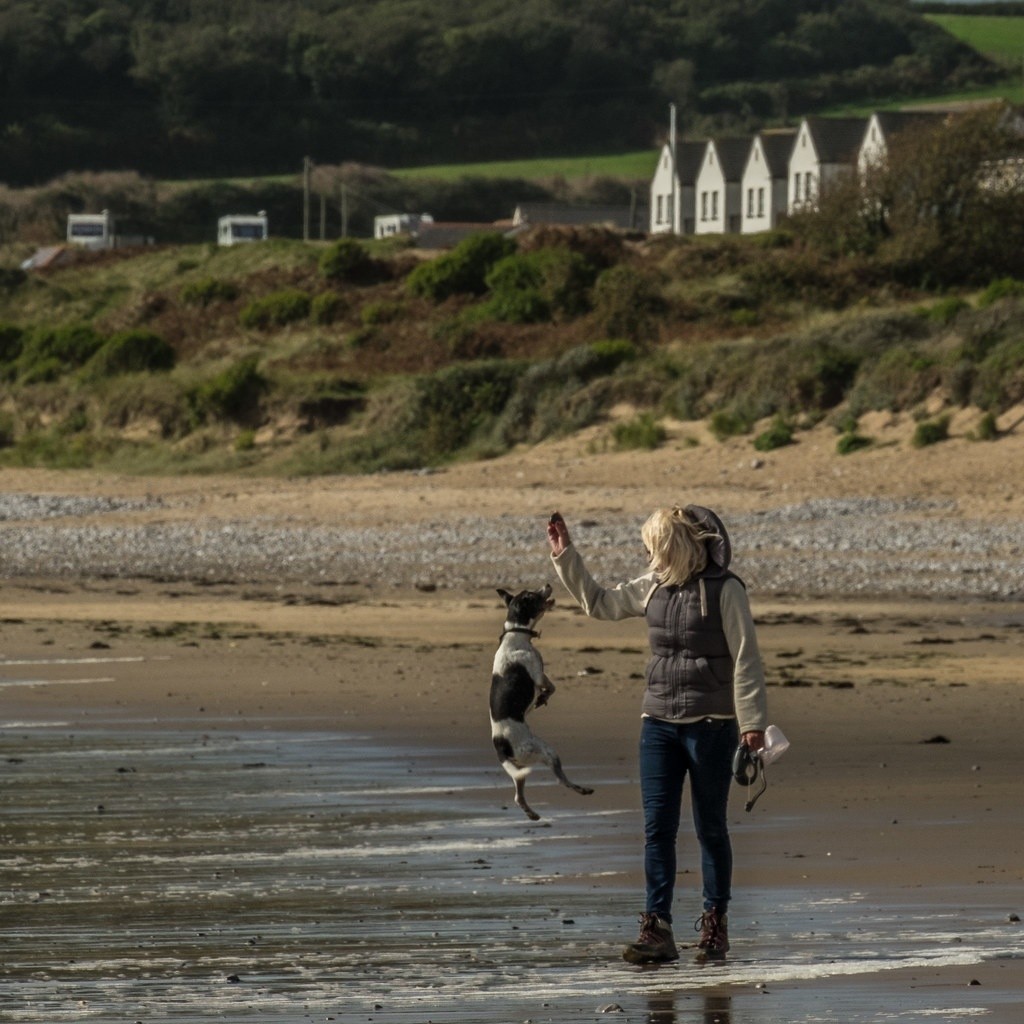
490 582 595 821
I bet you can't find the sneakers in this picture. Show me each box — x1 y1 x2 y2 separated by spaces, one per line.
694 907 730 960
624 914 679 964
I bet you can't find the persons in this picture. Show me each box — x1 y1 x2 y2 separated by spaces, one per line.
547 504 769 963
